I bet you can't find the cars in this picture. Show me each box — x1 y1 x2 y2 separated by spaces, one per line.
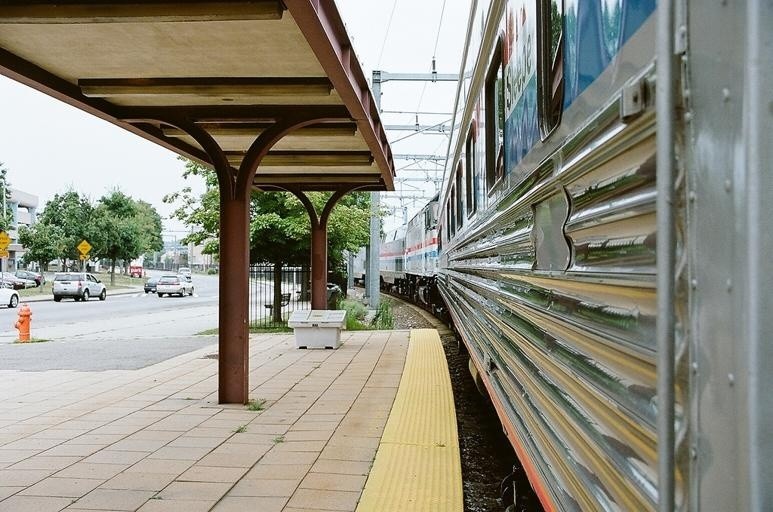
156 273 195 298
52 271 107 302
144 278 159 294
0 269 46 308
175 267 192 280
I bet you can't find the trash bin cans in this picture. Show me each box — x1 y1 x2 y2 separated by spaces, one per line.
327 283 341 309
130 266 143 278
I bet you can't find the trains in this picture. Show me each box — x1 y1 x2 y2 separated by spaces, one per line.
352 1 771 510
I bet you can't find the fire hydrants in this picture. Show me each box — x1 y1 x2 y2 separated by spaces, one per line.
14 302 33 342
